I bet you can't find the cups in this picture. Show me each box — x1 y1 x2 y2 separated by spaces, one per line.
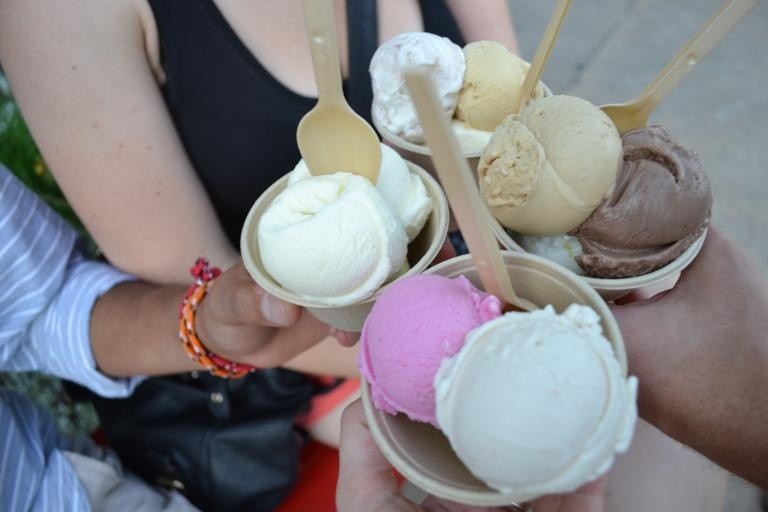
476 185 708 302
242 159 449 334
371 80 553 234
358 251 630 508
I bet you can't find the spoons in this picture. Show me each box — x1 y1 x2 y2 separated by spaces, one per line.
297 1 382 186
596 0 760 135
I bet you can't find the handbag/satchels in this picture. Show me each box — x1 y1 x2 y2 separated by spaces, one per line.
59 365 323 512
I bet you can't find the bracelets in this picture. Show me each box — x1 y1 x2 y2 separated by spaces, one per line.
176 252 261 382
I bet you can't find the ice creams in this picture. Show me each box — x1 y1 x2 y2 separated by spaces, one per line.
256 31 713 493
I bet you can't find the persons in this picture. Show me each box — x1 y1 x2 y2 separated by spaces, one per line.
0 161 365 511
0 1 531 389
332 394 618 510
594 220 766 490
